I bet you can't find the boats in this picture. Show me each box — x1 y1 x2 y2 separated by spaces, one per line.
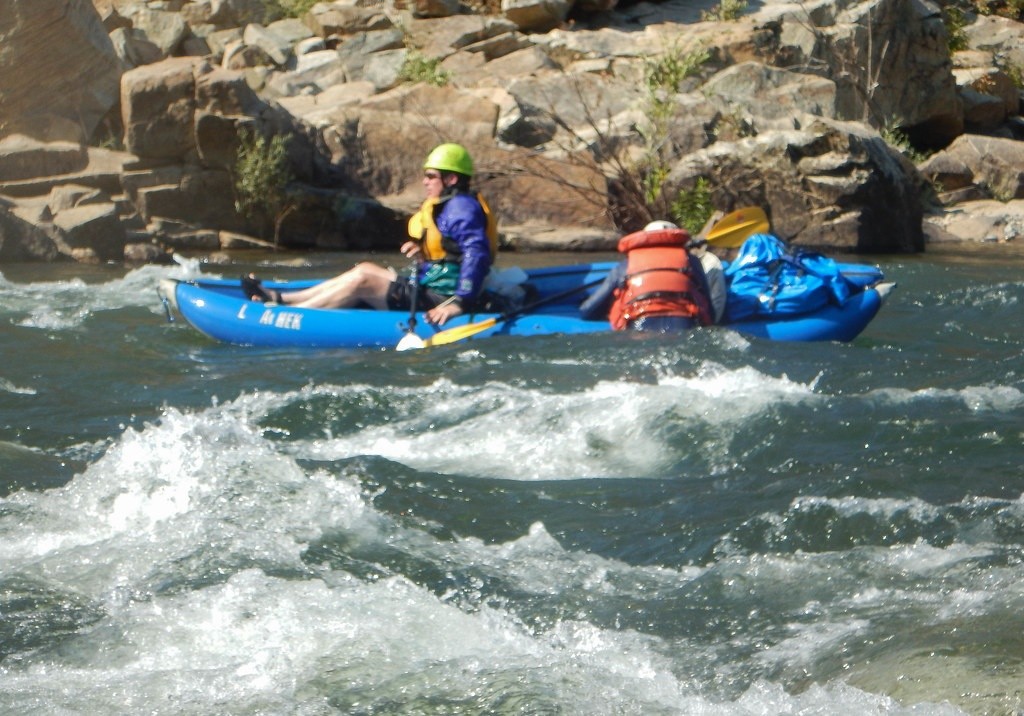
156 232 899 351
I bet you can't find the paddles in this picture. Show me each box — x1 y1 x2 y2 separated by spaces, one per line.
421 206 771 349
394 253 426 352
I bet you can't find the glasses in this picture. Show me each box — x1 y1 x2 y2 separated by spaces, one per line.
424 174 443 179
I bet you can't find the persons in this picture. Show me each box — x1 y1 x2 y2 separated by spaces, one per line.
579 220 717 330
240 142 498 325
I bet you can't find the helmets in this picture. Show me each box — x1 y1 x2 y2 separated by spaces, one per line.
423 143 474 177
642 220 679 230
